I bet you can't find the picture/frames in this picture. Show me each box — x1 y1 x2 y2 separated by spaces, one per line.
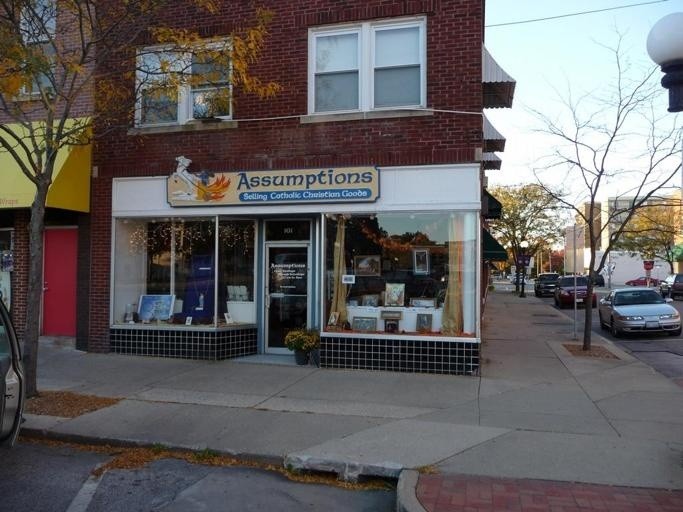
384 319 399 331
224 312 233 323
348 298 359 306
328 311 340 326
341 274 356 284
353 255 381 277
410 297 437 308
361 294 379 307
412 247 431 276
352 316 377 331
383 281 407 307
380 310 403 320
416 314 432 331
186 316 192 325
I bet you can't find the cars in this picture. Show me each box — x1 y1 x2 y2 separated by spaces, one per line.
587 274 604 286
554 274 598 309
512 273 526 285
534 273 559 297
658 273 683 299
599 287 682 337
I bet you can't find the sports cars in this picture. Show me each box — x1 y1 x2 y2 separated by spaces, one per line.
624 277 660 286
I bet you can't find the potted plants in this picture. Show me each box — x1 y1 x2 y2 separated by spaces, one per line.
286 325 321 365
